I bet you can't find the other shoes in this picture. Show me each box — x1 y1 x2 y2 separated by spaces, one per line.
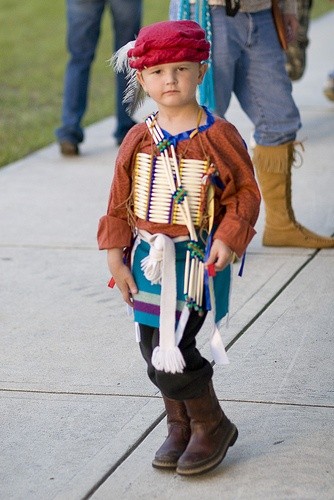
59 137 79 156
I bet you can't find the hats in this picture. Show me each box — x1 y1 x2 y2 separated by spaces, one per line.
127 20 210 72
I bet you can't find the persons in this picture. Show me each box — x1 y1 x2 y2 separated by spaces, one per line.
169 0 334 249
55 0 145 156
96 20 261 477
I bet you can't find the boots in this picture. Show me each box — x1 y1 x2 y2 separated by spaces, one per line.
176 378 238 477
251 139 334 248
152 391 191 470
